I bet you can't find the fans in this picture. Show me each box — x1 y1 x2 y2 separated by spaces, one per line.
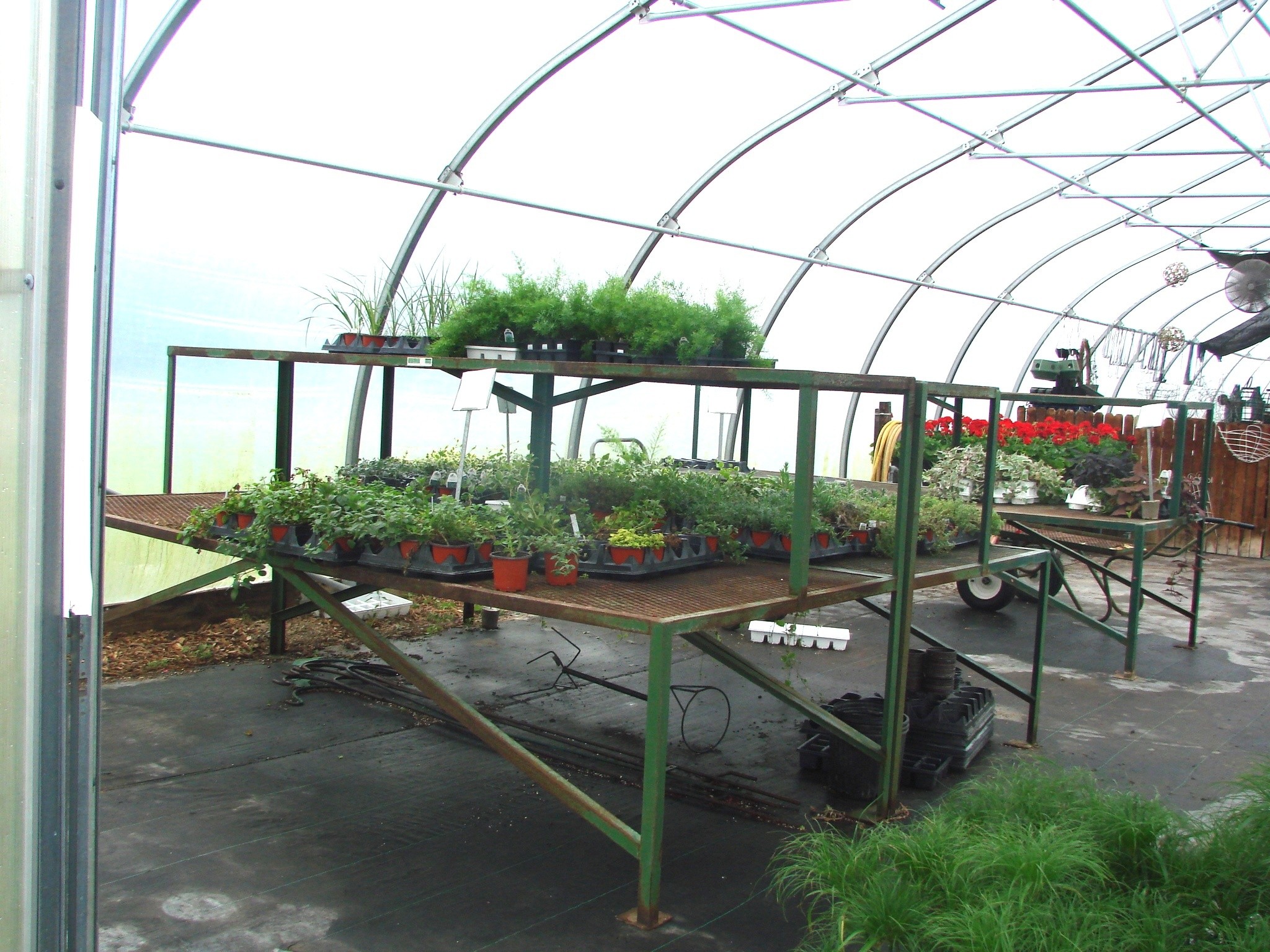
1224 250 1270 313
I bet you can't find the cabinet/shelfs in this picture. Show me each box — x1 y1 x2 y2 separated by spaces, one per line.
543 363 1050 826
100 353 911 931
969 393 1214 676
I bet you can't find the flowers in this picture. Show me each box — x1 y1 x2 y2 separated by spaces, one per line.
923 416 1134 474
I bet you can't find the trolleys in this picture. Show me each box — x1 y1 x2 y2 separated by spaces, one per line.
927 485 1256 626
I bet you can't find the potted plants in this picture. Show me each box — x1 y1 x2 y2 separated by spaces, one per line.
296 247 760 367
175 425 1062 600
1065 446 1132 511
